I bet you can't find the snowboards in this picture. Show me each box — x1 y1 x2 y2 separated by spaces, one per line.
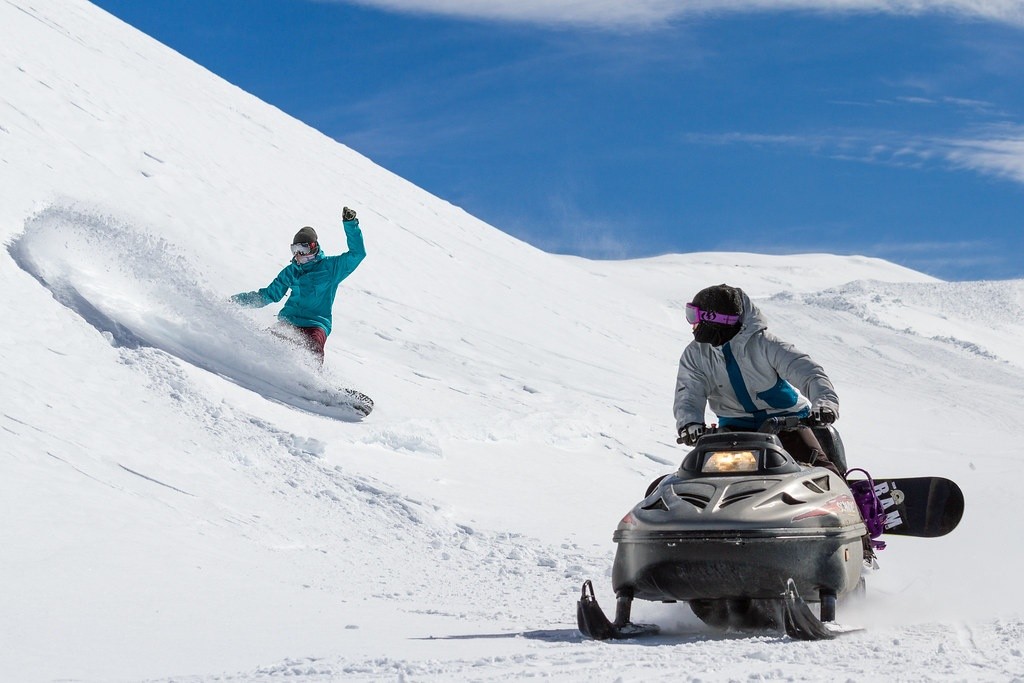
277 379 376 418
645 476 964 537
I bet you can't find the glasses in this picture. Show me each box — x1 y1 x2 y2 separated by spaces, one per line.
290 242 318 255
686 303 701 324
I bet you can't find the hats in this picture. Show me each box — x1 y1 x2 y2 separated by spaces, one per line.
692 283 743 344
292 226 318 245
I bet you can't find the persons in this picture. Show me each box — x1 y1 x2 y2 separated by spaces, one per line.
228 207 366 368
674 283 879 572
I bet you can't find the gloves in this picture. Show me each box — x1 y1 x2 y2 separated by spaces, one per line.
805 406 835 429
342 207 356 220
678 422 706 446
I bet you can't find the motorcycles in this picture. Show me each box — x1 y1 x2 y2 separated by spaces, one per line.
576 409 884 643
